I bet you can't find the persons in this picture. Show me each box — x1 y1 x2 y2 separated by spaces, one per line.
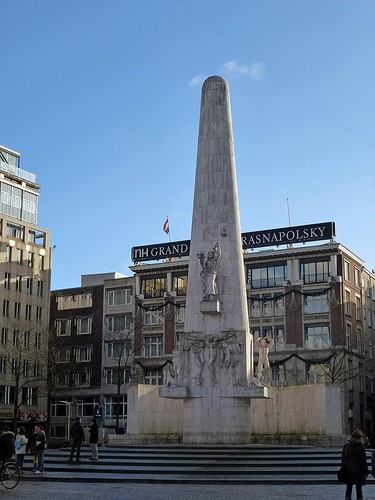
70 417 85 462
255 336 275 381
88 419 98 461
199 246 221 301
340 429 368 500
194 340 241 386
0 424 46 474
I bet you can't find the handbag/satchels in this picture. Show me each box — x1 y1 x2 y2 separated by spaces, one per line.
337 466 347 483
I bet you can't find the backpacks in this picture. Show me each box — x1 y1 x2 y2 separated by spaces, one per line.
15 434 24 450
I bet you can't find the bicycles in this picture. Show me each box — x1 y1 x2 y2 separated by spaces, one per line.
0 458 20 489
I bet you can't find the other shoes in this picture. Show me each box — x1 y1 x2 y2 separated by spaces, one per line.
89 458 98 461
76 459 80 463
67 459 73 463
15 469 45 475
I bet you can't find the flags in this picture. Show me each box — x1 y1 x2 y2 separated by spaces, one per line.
163 219 169 233
